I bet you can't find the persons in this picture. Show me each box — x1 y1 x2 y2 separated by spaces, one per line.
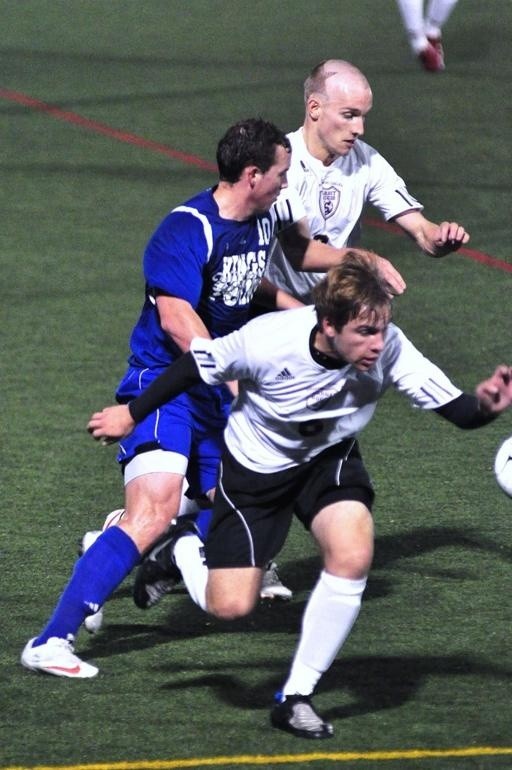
82 251 510 739
259 55 473 607
18 116 311 686
389 0 467 75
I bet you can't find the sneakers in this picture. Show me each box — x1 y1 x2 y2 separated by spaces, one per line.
76 529 111 636
269 692 335 741
414 26 446 73
260 561 294 607
133 514 201 610
19 635 101 681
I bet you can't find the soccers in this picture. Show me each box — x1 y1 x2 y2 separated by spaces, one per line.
494 437 511 495
